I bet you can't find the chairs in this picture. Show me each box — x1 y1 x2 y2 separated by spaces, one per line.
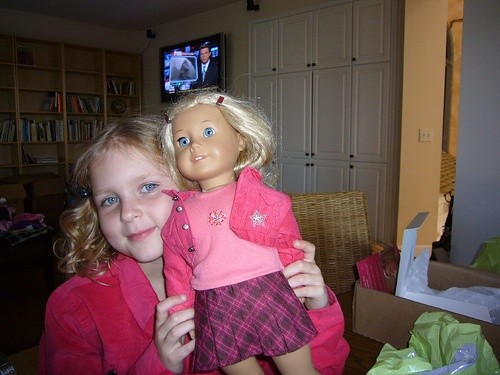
289 190 371 300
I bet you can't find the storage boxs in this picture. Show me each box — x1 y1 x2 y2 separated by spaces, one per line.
351 256 500 356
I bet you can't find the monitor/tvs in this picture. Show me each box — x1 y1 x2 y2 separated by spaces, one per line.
159 31 227 104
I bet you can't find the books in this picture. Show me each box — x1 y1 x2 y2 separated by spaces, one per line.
20 118 63 142
0 120 15 142
67 119 104 141
22 145 59 164
45 92 61 112
107 79 135 95
67 96 102 112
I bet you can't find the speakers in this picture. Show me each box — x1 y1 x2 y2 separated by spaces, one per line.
146 29 156 39
246 0 260 12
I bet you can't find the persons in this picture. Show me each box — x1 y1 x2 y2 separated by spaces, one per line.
38 115 350 375
192 45 218 89
161 92 319 375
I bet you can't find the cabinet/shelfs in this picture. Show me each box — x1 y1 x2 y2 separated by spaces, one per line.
0 34 143 181
0 222 61 359
